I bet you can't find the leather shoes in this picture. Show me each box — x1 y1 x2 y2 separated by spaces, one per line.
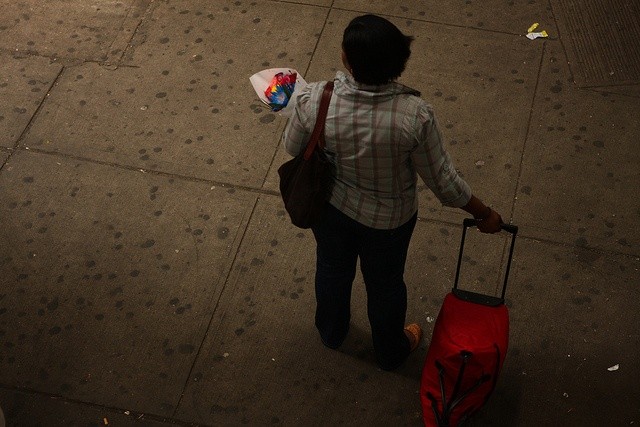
404 323 421 351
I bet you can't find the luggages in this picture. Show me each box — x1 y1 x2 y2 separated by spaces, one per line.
420 218 518 427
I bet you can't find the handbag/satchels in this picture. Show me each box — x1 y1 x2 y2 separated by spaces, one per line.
278 81 334 229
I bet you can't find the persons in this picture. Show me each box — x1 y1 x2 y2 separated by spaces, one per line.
280 14 501 371
